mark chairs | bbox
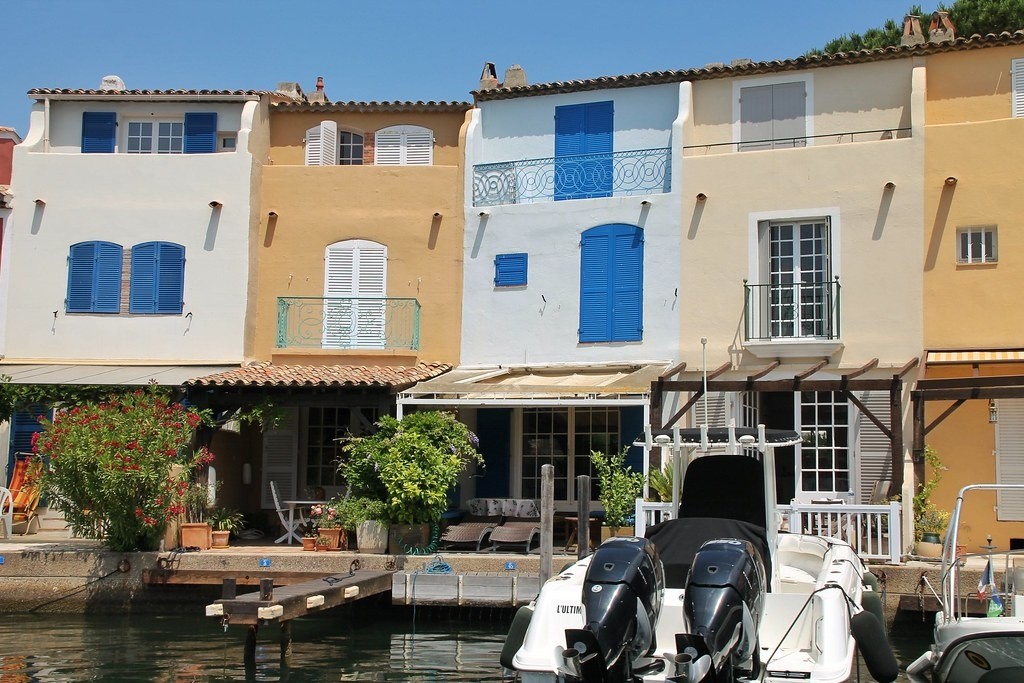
[270,480,310,544]
[2,452,44,536]
[488,521,540,554]
[0,486,12,539]
[438,522,498,553]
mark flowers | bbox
[310,496,344,528]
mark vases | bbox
[317,527,341,551]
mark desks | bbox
[564,517,597,551]
[283,500,328,547]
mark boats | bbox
[499,337,872,683]
[932,483,1024,683]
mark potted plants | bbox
[918,502,951,545]
[333,409,487,553]
[342,493,390,553]
[179,480,224,551]
[202,505,246,549]
[588,437,649,544]
[301,532,317,551]
[315,537,331,551]
